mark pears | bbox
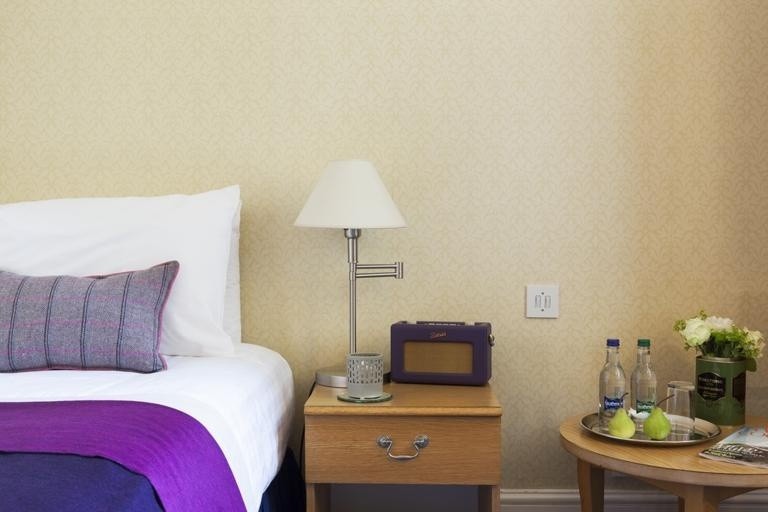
[609,392,636,439]
[643,393,674,440]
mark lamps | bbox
[291,159,410,388]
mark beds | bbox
[0,342,295,512]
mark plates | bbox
[580,413,722,446]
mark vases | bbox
[694,355,747,428]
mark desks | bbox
[559,412,768,512]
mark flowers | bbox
[674,310,765,360]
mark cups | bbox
[665,379,696,437]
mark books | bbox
[698,426,768,469]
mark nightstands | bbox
[303,382,503,512]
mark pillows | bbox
[0,260,180,374]
[0,184,242,356]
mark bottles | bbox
[596,337,659,434]
